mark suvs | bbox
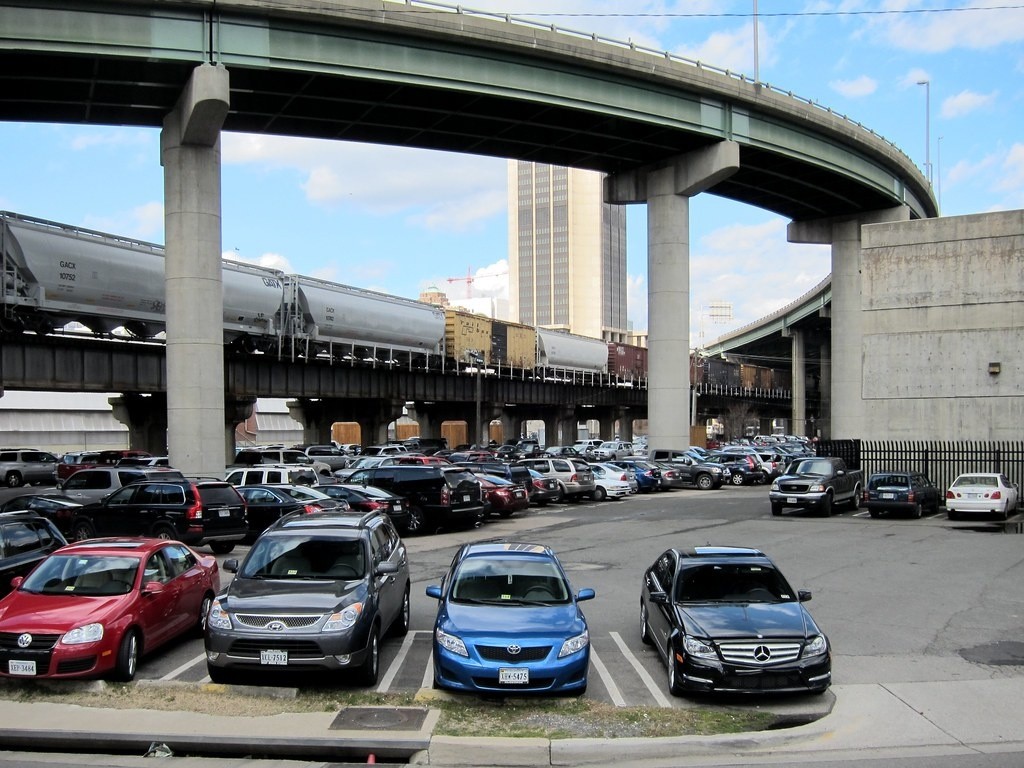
[204,503,412,686]
[0,509,70,599]
[338,463,485,533]
[37,466,186,507]
[517,458,597,503]
[67,476,248,554]
[458,462,534,495]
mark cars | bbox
[0,536,219,682]
[945,472,1019,519]
[529,469,561,507]
[864,472,941,518]
[426,540,595,698]
[0,437,587,483]
[473,473,529,517]
[313,485,411,529]
[588,433,816,500]
[640,543,831,699]
[234,484,350,538]
[1,495,85,516]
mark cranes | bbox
[446,265,508,300]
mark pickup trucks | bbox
[770,456,865,516]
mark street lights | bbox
[918,79,930,180]
